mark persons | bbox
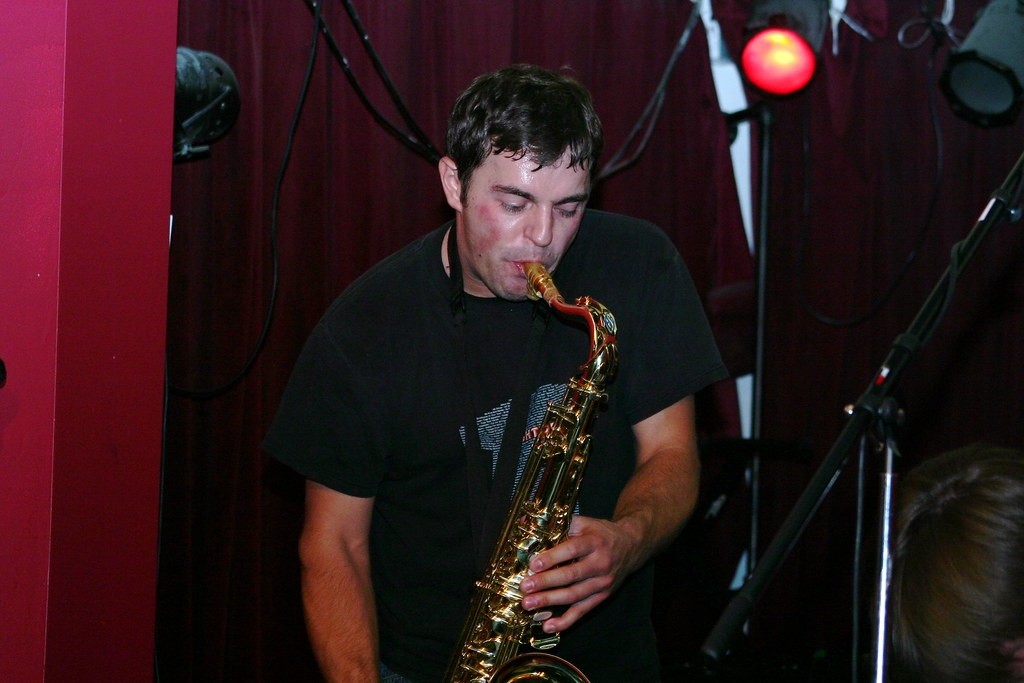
[268,64,722,683]
[890,441,1024,683]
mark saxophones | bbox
[438,263,621,683]
